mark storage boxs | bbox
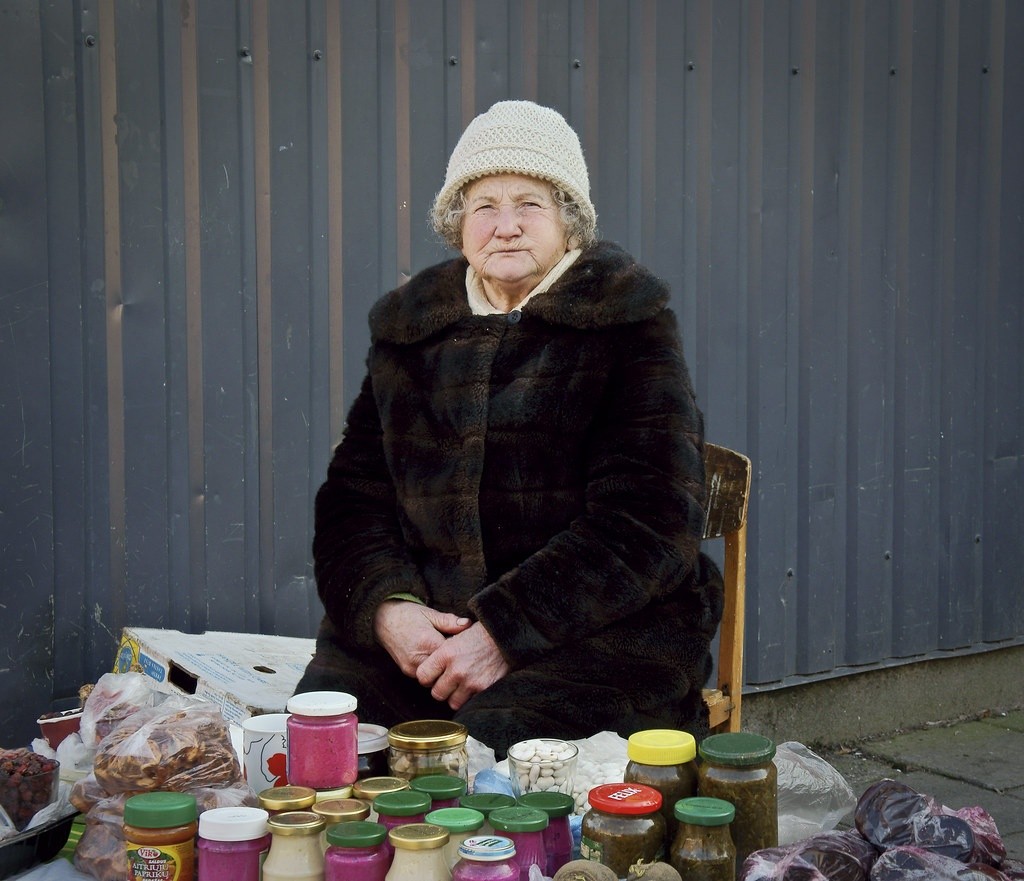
[114,626,315,781]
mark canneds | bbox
[122,692,778,881]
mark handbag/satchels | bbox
[753,741,1014,880]
[52,669,257,877]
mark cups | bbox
[243,713,292,794]
[508,738,579,797]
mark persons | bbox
[281,100,726,754]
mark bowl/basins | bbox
[37,707,83,751]
[0,759,61,832]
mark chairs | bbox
[701,441,752,735]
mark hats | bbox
[430,98,596,249]
